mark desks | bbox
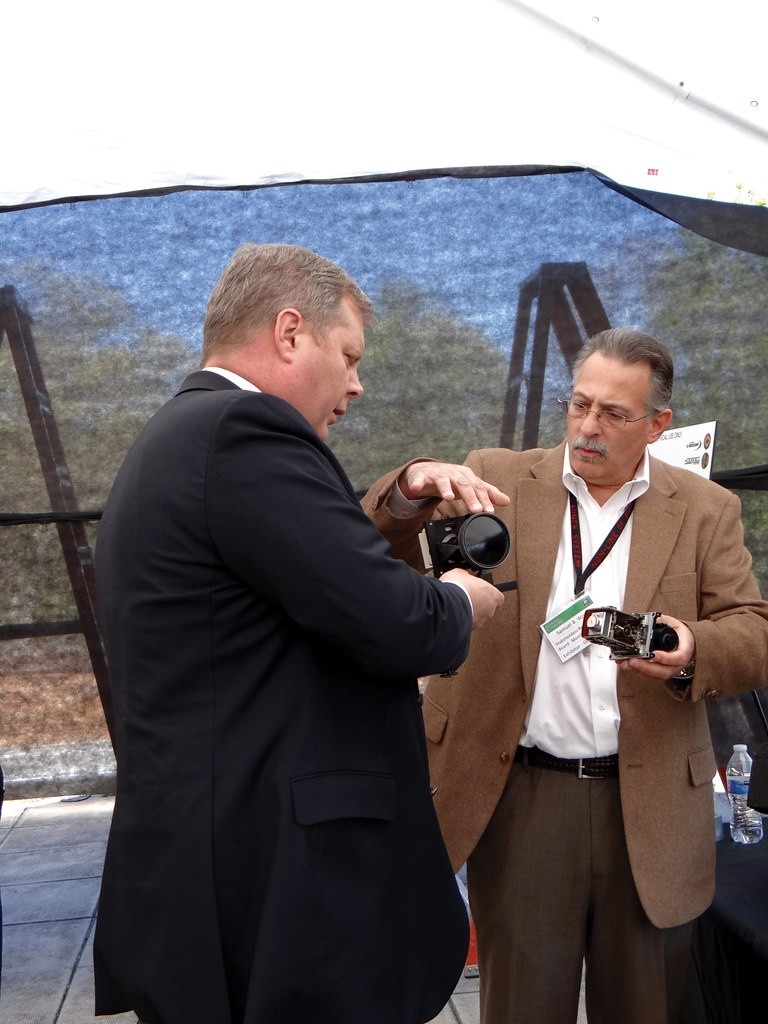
[686,805,768,1024]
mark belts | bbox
[514,746,620,780]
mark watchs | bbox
[676,665,692,678]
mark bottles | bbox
[726,744,763,844]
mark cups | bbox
[715,815,724,841]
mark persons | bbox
[92,242,504,1023]
[361,328,768,1024]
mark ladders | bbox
[2,294,122,783]
[497,256,615,451]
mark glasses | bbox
[557,385,655,430]
